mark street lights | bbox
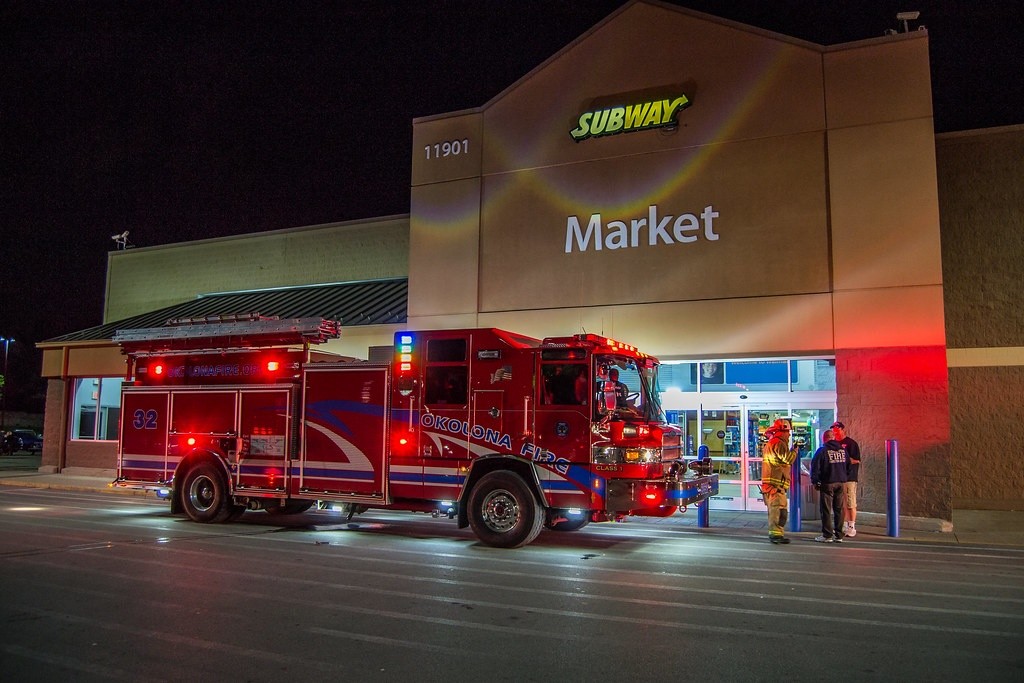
[0,337,15,366]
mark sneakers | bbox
[845,526,856,537]
[815,536,833,543]
[833,526,849,533]
[833,538,842,543]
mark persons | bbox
[701,363,723,384]
[762,420,798,544]
[811,430,847,542]
[830,422,861,537]
[600,368,628,407]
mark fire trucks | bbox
[108,316,720,549]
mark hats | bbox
[830,422,844,429]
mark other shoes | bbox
[770,537,790,544]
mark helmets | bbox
[769,420,792,430]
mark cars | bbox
[1,429,43,453]
